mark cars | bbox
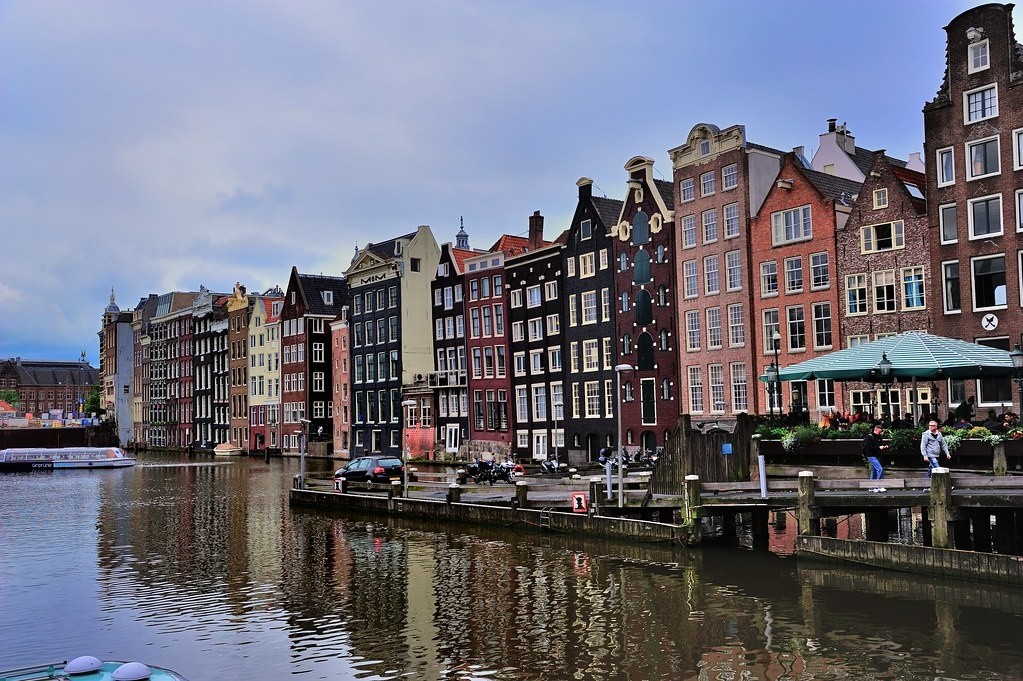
[334,454,404,491]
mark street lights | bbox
[765,359,776,418]
[1008,341,1023,429]
[402,400,417,497]
[878,350,892,429]
[299,418,312,490]
[554,402,565,464]
[616,365,634,510]
[771,330,784,416]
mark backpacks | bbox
[861,434,874,452]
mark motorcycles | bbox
[465,453,518,487]
[600,445,663,472]
[541,453,563,475]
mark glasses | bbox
[929,425,937,427]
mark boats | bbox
[213,443,244,456]
[0,447,138,470]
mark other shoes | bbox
[878,488,887,492]
[869,489,879,492]
[923,488,930,492]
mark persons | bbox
[491,453,496,463]
[861,425,889,493]
[920,420,955,494]
[818,400,1020,434]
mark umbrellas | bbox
[760,331,1015,431]
